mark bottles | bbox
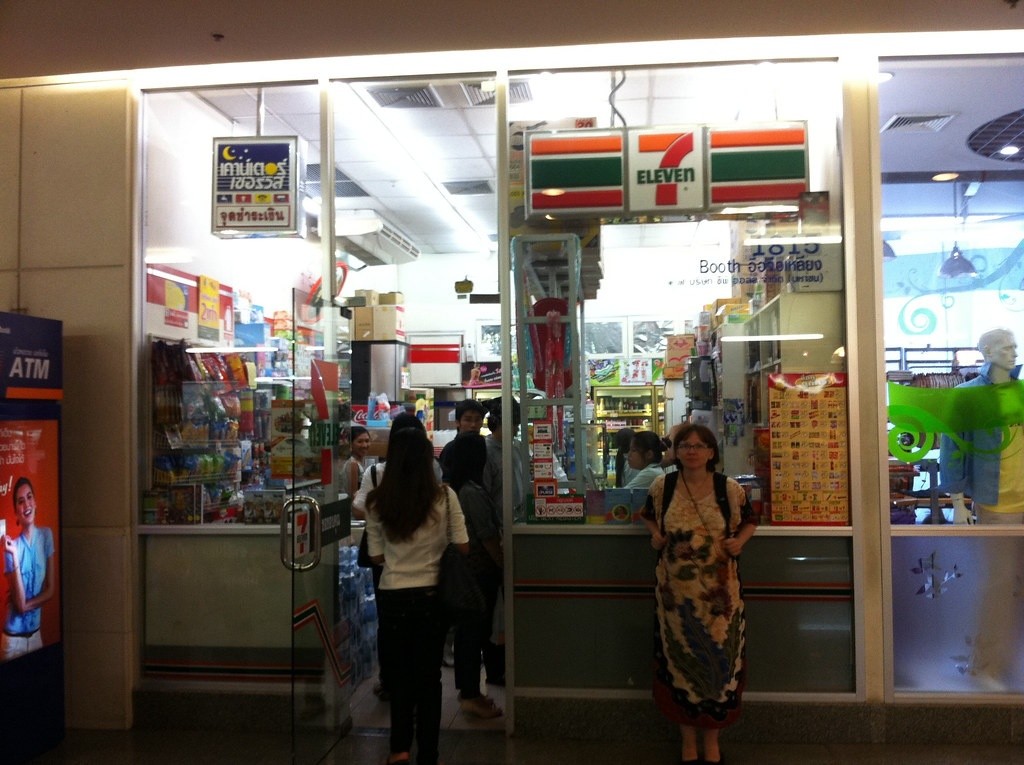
[338,545,379,679]
[599,398,644,410]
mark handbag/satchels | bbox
[358,466,382,568]
[434,543,484,617]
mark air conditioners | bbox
[317,209,420,266]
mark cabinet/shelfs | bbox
[884,347,985,525]
[743,290,849,475]
[709,323,753,478]
[144,332,258,525]
[513,234,587,525]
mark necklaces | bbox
[685,477,706,498]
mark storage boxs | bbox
[379,292,405,305]
[403,387,434,445]
[729,190,844,302]
[355,290,379,306]
[347,306,373,341]
[234,304,316,381]
[374,305,407,343]
[508,115,605,301]
[653,357,665,386]
[664,297,759,378]
[589,359,620,386]
[619,358,652,387]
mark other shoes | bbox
[388,752,409,764]
[443,641,454,666]
[681,750,723,765]
[372,680,389,699]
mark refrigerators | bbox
[589,386,664,483]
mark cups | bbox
[275,385,290,400]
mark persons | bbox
[624,431,672,488]
[443,433,504,718]
[483,396,529,552]
[614,428,675,488]
[939,325,1024,692]
[352,412,442,702]
[640,423,759,765]
[439,399,488,496]
[364,428,469,764]
[342,426,371,493]
[1,476,55,660]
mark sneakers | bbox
[457,692,502,719]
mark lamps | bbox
[883,240,897,263]
[940,183,978,279]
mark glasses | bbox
[678,442,707,452]
